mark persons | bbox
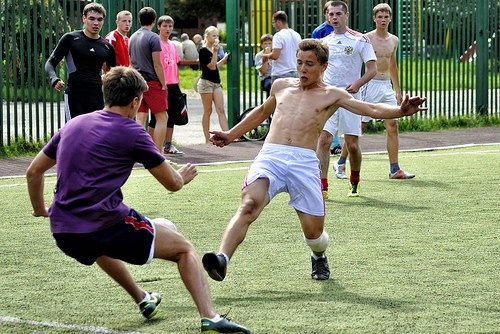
[128,7,173,164]
[193,35,204,51]
[148,15,179,154]
[217,45,224,62]
[268,11,301,82]
[333,3,415,179]
[197,26,241,143]
[316,1,378,199]
[202,39,428,281]
[256,34,274,92]
[45,3,116,121]
[312,1,342,154]
[26,66,250,334]
[181,34,199,60]
[172,37,183,60]
[102,11,132,74]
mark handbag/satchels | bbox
[178,85,189,125]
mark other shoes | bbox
[323,191,329,200]
[330,144,342,156]
[349,181,360,197]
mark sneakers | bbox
[202,251,228,281]
[310,255,330,280]
[333,161,348,179]
[200,313,250,334]
[163,146,184,157]
[139,291,162,318]
[389,169,415,179]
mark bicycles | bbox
[238,106,272,141]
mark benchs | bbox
[178,59,200,66]
